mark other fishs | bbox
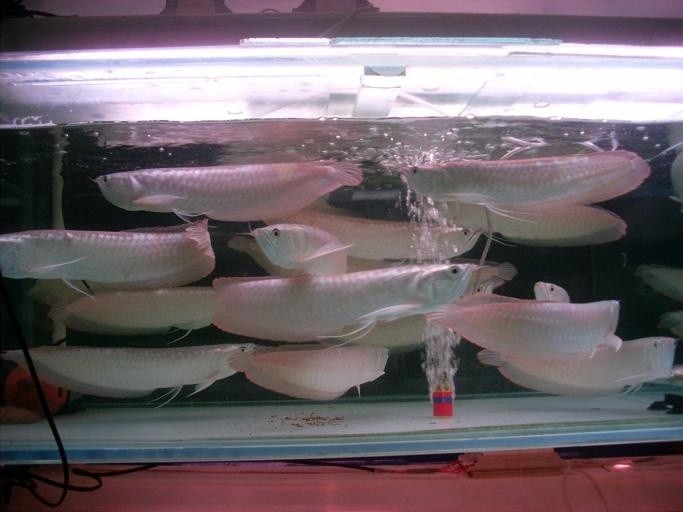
[211,261,518,353]
[93,158,364,230]
